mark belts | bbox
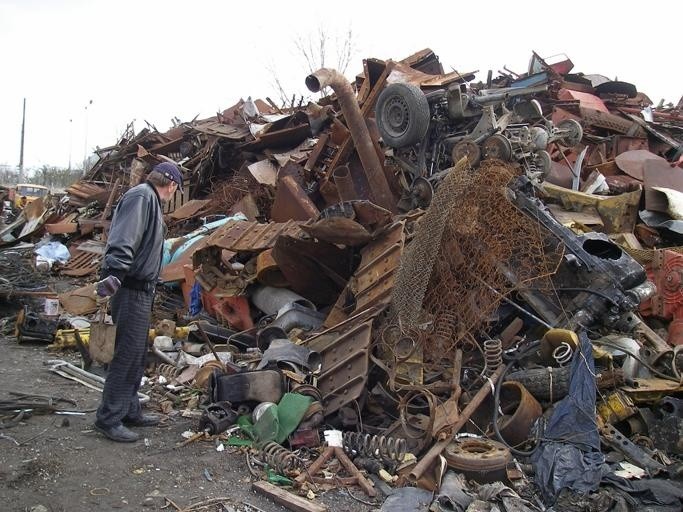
[124,275,158,297]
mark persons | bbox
[91,160,184,443]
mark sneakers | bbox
[93,419,141,443]
[124,409,162,428]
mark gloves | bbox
[92,274,122,305]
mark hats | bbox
[155,160,186,197]
